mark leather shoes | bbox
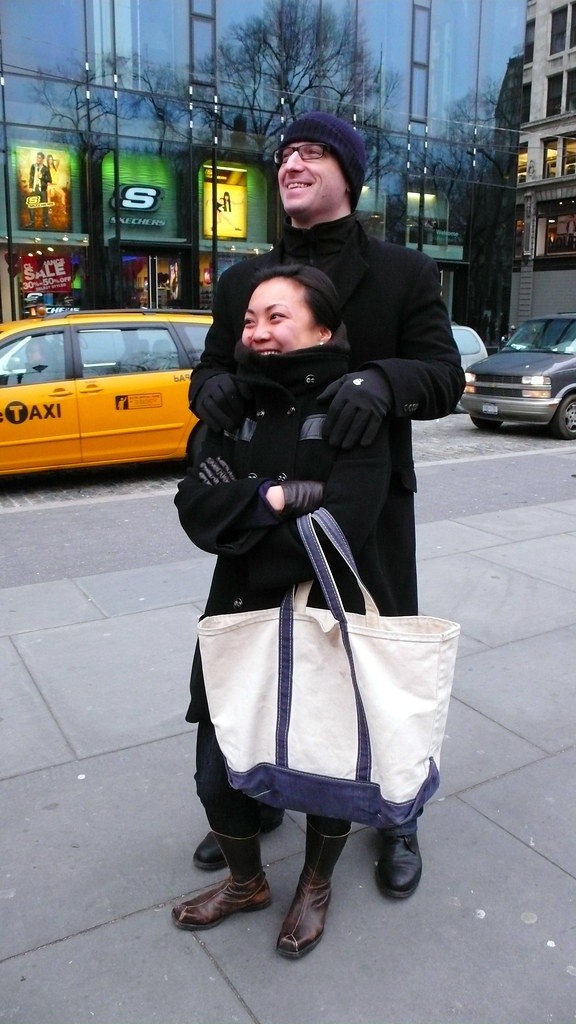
[194,814,284,872]
[375,835,422,898]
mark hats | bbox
[275,112,367,212]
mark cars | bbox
[0,308,213,480]
[449,321,490,416]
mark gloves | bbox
[280,480,327,520]
[316,367,395,452]
[195,373,253,434]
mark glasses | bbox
[274,142,330,165]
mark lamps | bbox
[62,235,68,241]
[83,236,88,242]
[230,245,234,250]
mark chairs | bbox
[113,340,149,372]
[145,339,173,370]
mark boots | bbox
[171,830,271,931]
[276,822,351,960]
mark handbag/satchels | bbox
[197,507,460,829]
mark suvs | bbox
[458,311,575,443]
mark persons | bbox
[187,109,467,901]
[167,264,389,959]
[211,191,243,232]
[24,152,69,229]
